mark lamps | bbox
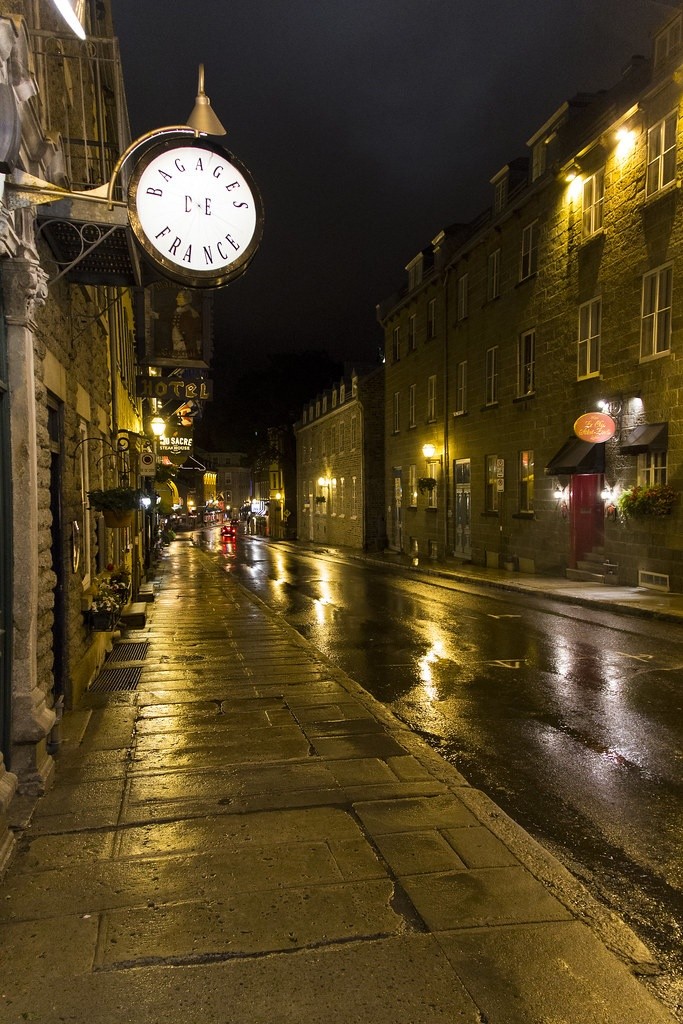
[316,477,330,490]
[276,492,285,504]
[553,487,565,499]
[149,415,166,442]
[185,61,227,138]
[139,488,152,506]
[595,388,642,408]
[422,443,445,473]
[600,487,611,503]
[563,164,580,182]
[152,490,161,505]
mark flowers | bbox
[625,483,677,507]
[85,578,130,614]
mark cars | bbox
[230,518,239,526]
[221,525,236,536]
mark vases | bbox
[92,611,112,629]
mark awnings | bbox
[619,422,668,454]
[545,436,606,475]
[154,480,180,504]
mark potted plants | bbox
[85,487,140,528]
[110,562,130,602]
